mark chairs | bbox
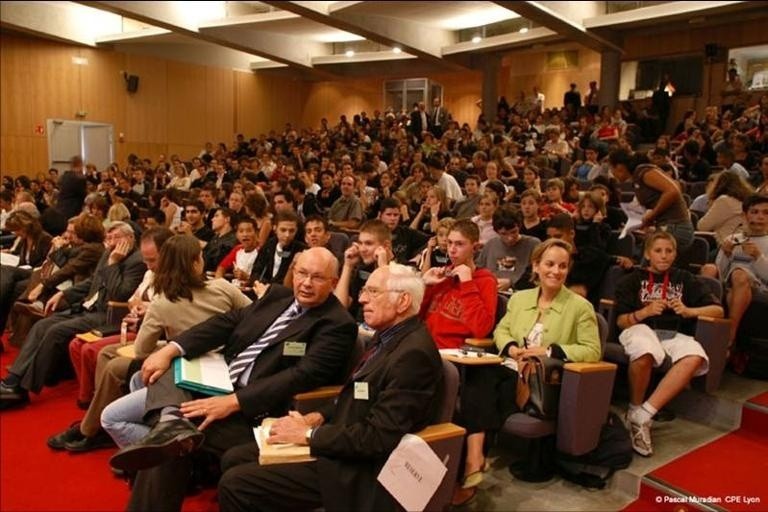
[106,181,733,512]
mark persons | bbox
[0,67,768,512]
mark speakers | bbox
[128,75,138,92]
[705,42,718,61]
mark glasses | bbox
[730,222,755,246]
[293,270,335,284]
[357,287,402,299]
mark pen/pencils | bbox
[523,337,527,349]
[233,261,238,271]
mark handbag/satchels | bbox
[514,354,563,422]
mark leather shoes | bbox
[0,389,30,411]
[12,301,45,319]
[47,427,86,450]
[65,433,116,454]
[461,459,490,489]
[110,418,204,470]
[1,381,25,400]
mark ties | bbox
[228,304,301,382]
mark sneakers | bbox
[626,410,653,456]
